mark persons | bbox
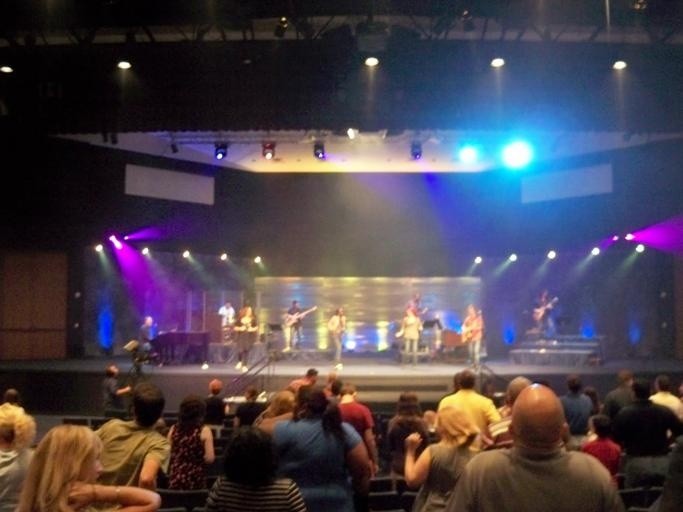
[216,300,236,328]
[327,306,348,368]
[374,372,683,512]
[404,292,426,319]
[239,296,255,319]
[458,304,484,370]
[530,288,558,354]
[136,315,155,353]
[283,301,309,351]
[393,307,422,367]
[232,307,258,373]
[1,364,380,512]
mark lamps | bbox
[213,128,422,160]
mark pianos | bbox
[157,331,207,365]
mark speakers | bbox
[71,217,109,245]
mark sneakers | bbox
[334,362,343,371]
[281,346,290,352]
[235,362,249,373]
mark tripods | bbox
[117,368,158,416]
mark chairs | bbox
[204,415,238,489]
[366,479,418,512]
[156,489,208,512]
[612,472,664,508]
[162,410,180,426]
[62,409,129,432]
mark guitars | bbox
[285,306,318,327]
[533,296,558,321]
[461,319,479,344]
